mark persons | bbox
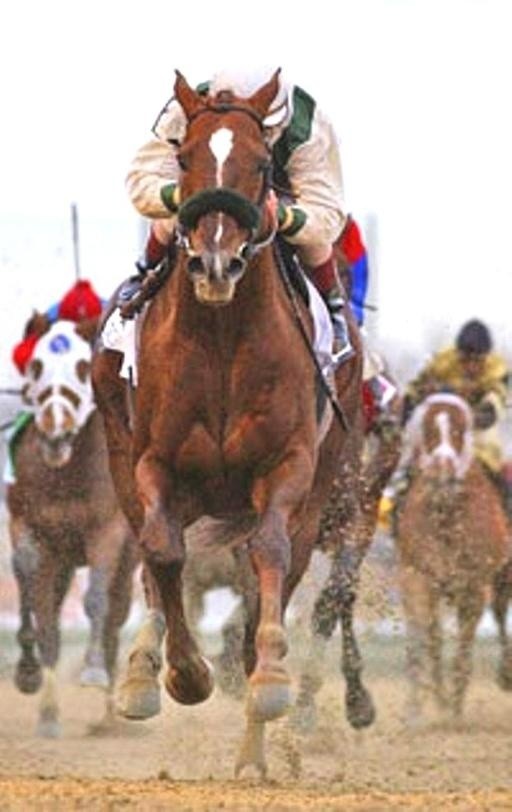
[11,279,509,528]
[125,71,371,330]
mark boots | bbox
[322,283,356,368]
[119,259,156,300]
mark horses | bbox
[90,67,365,790]
[187,369,404,731]
[4,306,133,737]
[387,378,512,733]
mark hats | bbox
[458,320,491,352]
[58,280,103,321]
